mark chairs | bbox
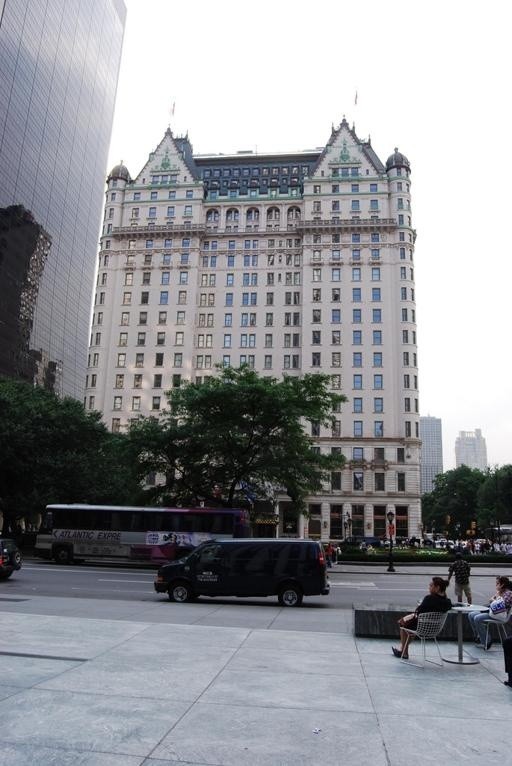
[397,609,445,668]
[482,599,512,650]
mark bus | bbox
[34,503,253,570]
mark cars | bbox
[339,533,469,550]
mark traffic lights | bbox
[445,515,450,525]
[467,530,475,536]
[443,531,448,535]
[471,521,476,528]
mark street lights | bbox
[489,519,495,542]
[456,522,461,553]
[455,527,458,539]
[386,509,396,573]
[344,522,347,541]
[346,518,353,543]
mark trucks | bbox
[497,524,512,544]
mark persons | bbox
[446,552,472,605]
[326,536,511,571]
[467,576,511,650]
[391,577,451,659]
[161,532,192,546]
[501,637,511,687]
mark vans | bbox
[155,538,330,607]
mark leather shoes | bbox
[392,645,408,659]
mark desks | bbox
[442,598,489,664]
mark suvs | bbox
[0,539,25,581]
